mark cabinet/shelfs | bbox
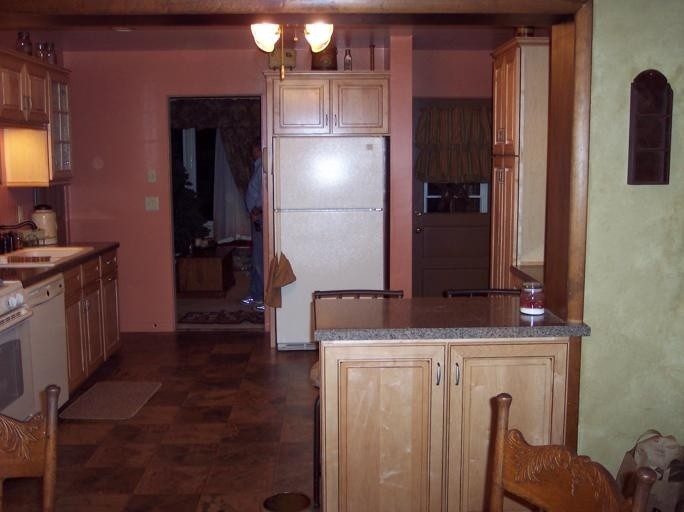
[490,46,549,297]
[325,343,569,512]
[273,79,389,134]
[5,70,74,185]
[65,250,122,393]
[1,52,50,125]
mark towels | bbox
[265,253,297,309]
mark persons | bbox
[238,130,265,313]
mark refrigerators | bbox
[273,136,386,351]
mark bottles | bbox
[343,49,352,71]
[0,204,58,255]
[15,31,58,65]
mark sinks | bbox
[1,255,66,269]
[4,245,94,256]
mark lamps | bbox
[251,23,336,81]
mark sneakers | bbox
[240,296,264,312]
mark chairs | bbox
[489,394,657,512]
[0,385,63,512]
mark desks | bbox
[179,246,238,299]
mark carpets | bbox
[178,310,265,324]
[58,380,163,421]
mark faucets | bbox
[1,219,38,231]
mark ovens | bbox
[0,304,35,422]
